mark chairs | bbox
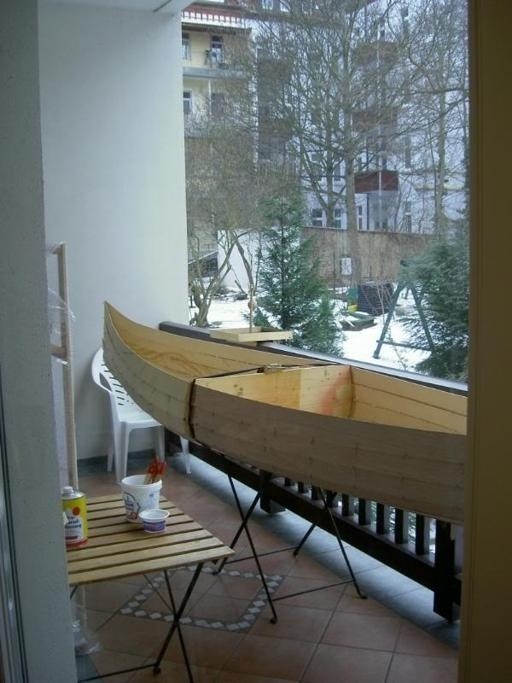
[91,344,191,484]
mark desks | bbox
[66,493,237,682]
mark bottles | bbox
[60,486,89,549]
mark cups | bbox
[120,474,162,524]
[138,508,169,532]
